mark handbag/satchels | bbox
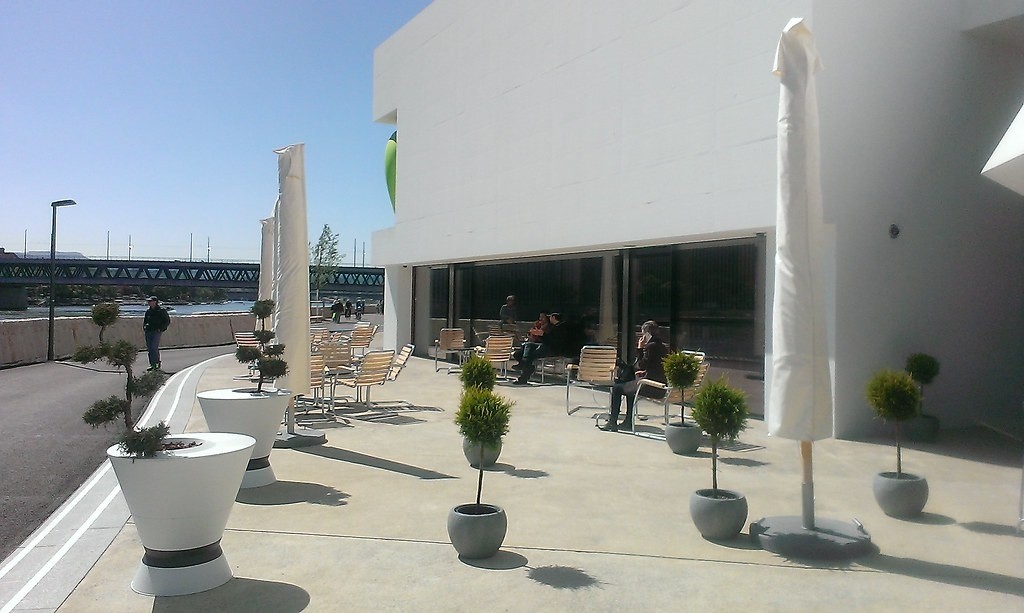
[616,356,637,381]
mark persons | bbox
[142,296,170,371]
[580,306,598,329]
[330,297,384,324]
[597,321,668,432]
[500,296,568,385]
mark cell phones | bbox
[642,332,652,345]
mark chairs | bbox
[232,318,414,429]
[433,328,710,439]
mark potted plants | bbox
[660,348,707,455]
[445,382,516,562]
[866,367,930,519]
[74,299,256,598]
[195,300,292,488]
[902,353,941,441]
[688,372,750,543]
[457,351,503,468]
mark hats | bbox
[145,296,158,301]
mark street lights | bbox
[48,200,75,362]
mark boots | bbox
[617,394,635,431]
[600,388,621,432]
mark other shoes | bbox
[511,361,527,370]
[147,362,161,371]
[514,377,529,385]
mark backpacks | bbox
[158,308,170,332]
